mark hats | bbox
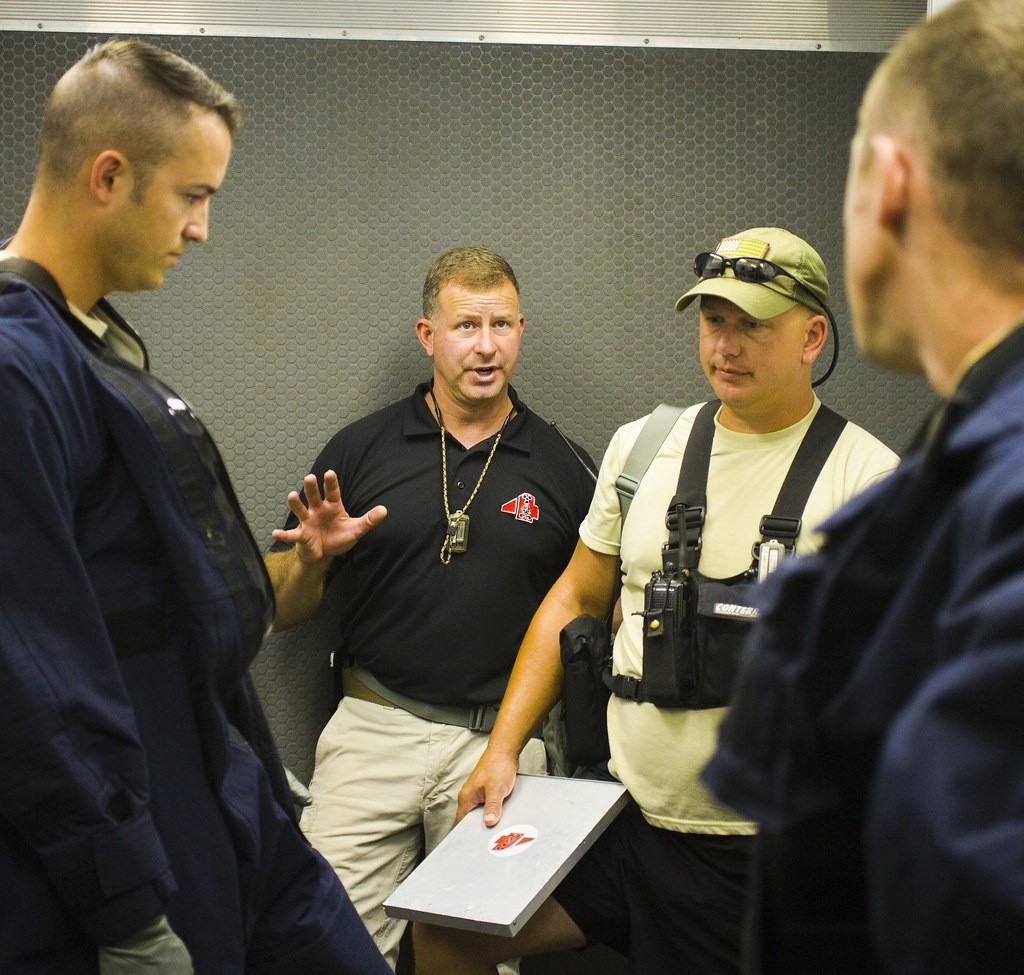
[674,227,830,321]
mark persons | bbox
[697,0,1024,975]
[456,227,901,975]
[2,40,393,975]
[263,248,623,975]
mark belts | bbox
[342,670,545,738]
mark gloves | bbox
[98,914,193,975]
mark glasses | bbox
[693,252,824,306]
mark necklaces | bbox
[430,389,516,565]
